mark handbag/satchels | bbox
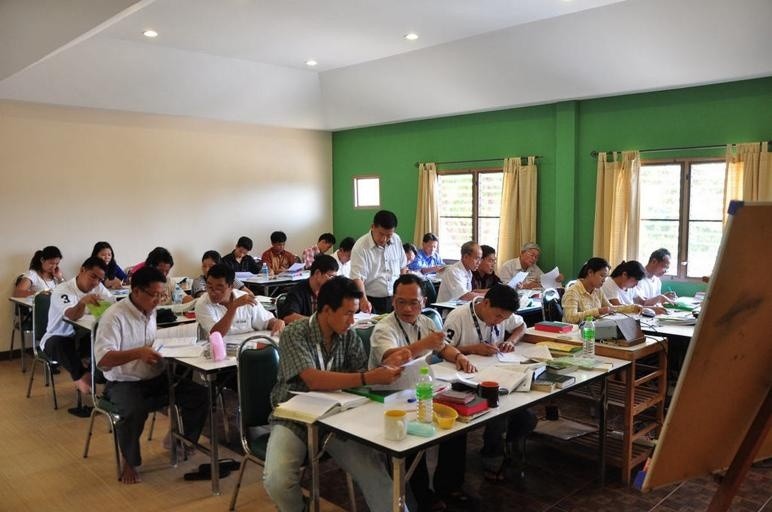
[157,308,177,323]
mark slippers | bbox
[184,458,240,480]
[483,468,508,486]
[68,404,94,417]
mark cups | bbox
[477,381,499,407]
[384,409,407,438]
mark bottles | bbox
[416,367,433,424]
[261,262,270,283]
[582,315,596,358]
[173,285,183,317]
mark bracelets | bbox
[58,278,64,282]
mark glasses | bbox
[139,287,167,300]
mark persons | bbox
[127,231,574,342]
[601,260,676,315]
[442,284,538,493]
[93,265,210,484]
[264,276,410,512]
[350,209,407,314]
[39,258,118,396]
[369,273,483,512]
[634,248,680,304]
[14,247,66,375]
[91,242,127,290]
[561,258,643,325]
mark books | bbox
[86,298,113,319]
[272,340,582,427]
[153,323,202,360]
[653,291,706,327]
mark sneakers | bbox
[425,490,474,512]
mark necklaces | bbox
[39,273,57,288]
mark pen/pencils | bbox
[667,286,672,294]
[377,364,407,376]
[156,345,163,352]
[429,327,452,344]
[484,341,504,357]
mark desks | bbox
[9,258,722,512]
